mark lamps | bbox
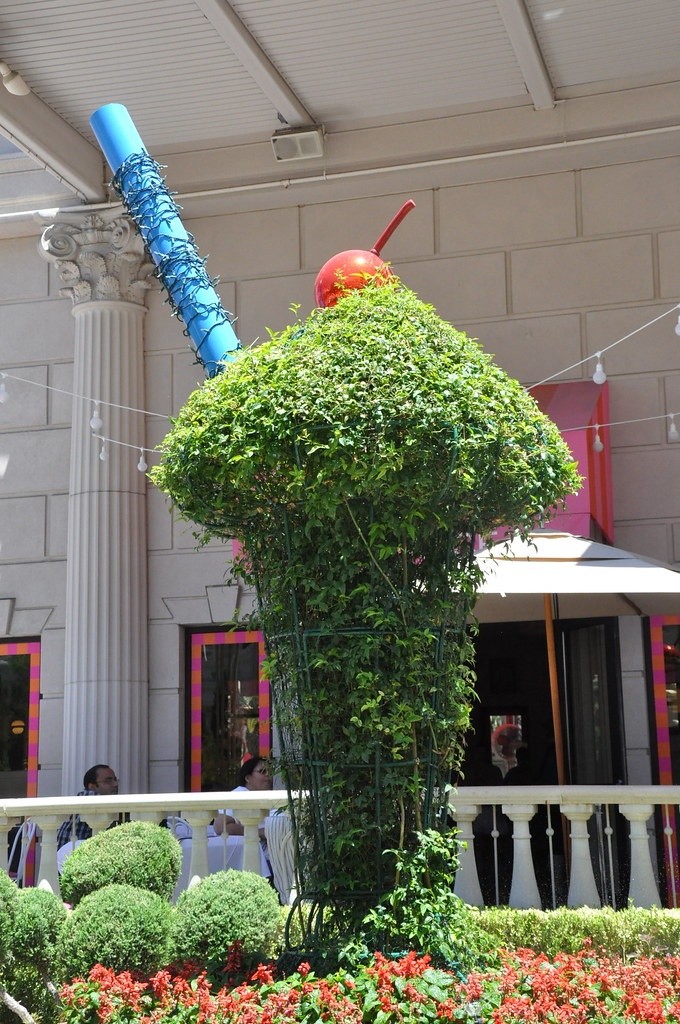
[272,124,325,162]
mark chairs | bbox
[266,814,315,907]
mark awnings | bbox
[230,380,615,578]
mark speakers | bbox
[270,125,324,162]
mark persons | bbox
[58,763,132,877]
[213,756,276,877]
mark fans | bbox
[492,723,528,769]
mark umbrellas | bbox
[415,524,680,883]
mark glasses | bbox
[253,769,273,775]
[94,777,120,784]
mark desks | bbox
[56,823,273,908]
